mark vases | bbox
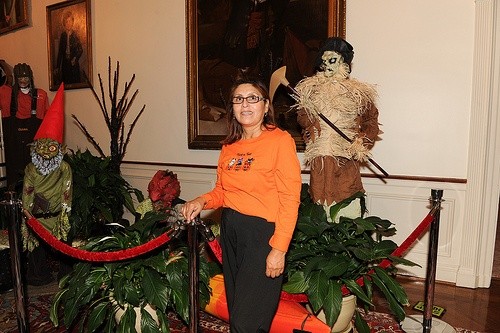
[108,288,159,333]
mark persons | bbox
[181,80,302,333]
[295,38,379,224]
[0,63,73,286]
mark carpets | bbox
[0,283,484,333]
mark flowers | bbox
[51,170,212,333]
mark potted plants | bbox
[279,183,423,333]
[63,147,145,248]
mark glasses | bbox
[230,95,265,103]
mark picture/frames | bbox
[46,0,94,91]
[0,0,29,34]
[185,0,346,152]
